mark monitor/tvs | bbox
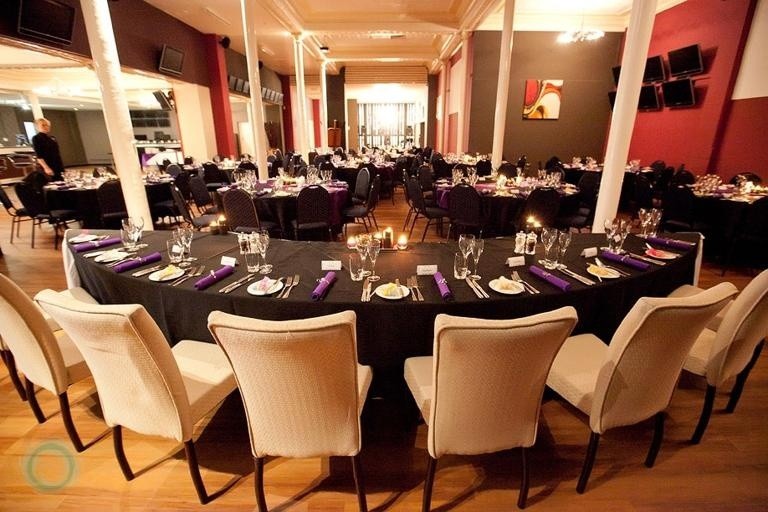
[611,65,621,88]
[608,92,616,112]
[237,79,243,91]
[643,55,666,83]
[661,78,696,108]
[262,88,283,102]
[637,85,660,110]
[667,43,704,78]
[228,75,236,90]
[152,90,173,112]
[16,0,77,49]
[157,42,187,77]
[243,81,249,92]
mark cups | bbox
[244,247,259,273]
[348,253,364,281]
[453,253,467,280]
[608,232,620,255]
[166,239,183,264]
[544,245,558,270]
[120,228,136,248]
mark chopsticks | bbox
[630,253,666,265]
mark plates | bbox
[586,267,620,279]
[488,278,524,295]
[247,279,283,296]
[149,270,185,282]
[645,250,677,259]
[94,253,128,263]
[375,284,409,300]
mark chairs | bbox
[219,146,530,178]
[165,166,181,179]
[33,289,238,505]
[223,190,270,232]
[204,168,219,191]
[445,183,482,239]
[14,183,63,248]
[409,187,448,242]
[0,286,101,402]
[404,306,579,512]
[545,281,740,494]
[682,268,768,446]
[190,178,214,213]
[347,167,372,229]
[343,175,381,235]
[403,181,440,236]
[516,187,560,232]
[667,284,764,414]
[171,185,218,231]
[30,190,86,251]
[208,310,372,512]
[214,143,528,179]
[0,273,112,453]
[0,186,30,246]
[293,185,332,241]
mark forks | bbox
[595,258,631,275]
[514,270,540,294]
[595,261,627,278]
[406,278,417,301]
[411,275,424,301]
[108,257,141,268]
[511,275,534,294]
[107,252,137,266]
[276,276,292,298]
[646,242,652,249]
[282,274,299,298]
[168,266,196,286]
[173,265,205,286]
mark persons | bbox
[32,117,65,176]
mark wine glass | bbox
[468,239,483,281]
[60,170,77,184]
[458,234,475,275]
[257,234,273,274]
[173,230,184,258]
[600,217,619,249]
[122,217,140,251]
[651,208,661,238]
[538,227,557,265]
[636,209,646,237]
[557,230,570,269]
[450,167,479,187]
[233,170,257,189]
[132,217,148,248]
[307,167,334,185]
[357,238,371,276]
[617,218,633,255]
[367,240,381,282]
[642,213,651,237]
[179,228,198,261]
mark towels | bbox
[310,270,336,301]
[433,271,451,300]
[645,237,691,252]
[193,265,234,290]
[113,251,162,272]
[256,190,269,196]
[600,250,650,271]
[73,237,121,253]
[529,265,571,291]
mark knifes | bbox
[558,268,592,286]
[472,279,489,299]
[225,276,255,293]
[367,283,372,301]
[564,269,596,284]
[219,273,255,293]
[465,277,483,298]
[361,279,368,302]
